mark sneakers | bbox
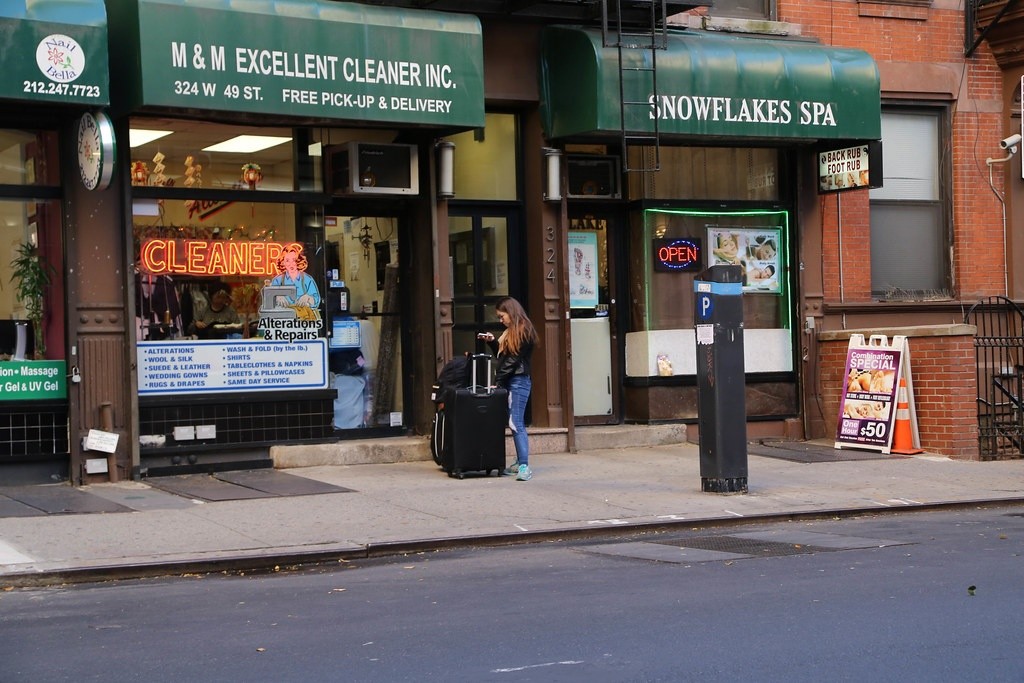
[504,460,519,475]
[516,464,532,481]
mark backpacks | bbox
[431,352,472,407]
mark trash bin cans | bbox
[45,453,70,483]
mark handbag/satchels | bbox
[430,408,445,465]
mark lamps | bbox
[434,138,456,199]
[541,146,564,203]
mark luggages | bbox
[444,353,508,477]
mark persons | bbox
[713,231,778,286]
[819,171,868,191]
[477,297,539,481]
[844,368,887,420]
[187,282,246,340]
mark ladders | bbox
[616,0,661,175]
